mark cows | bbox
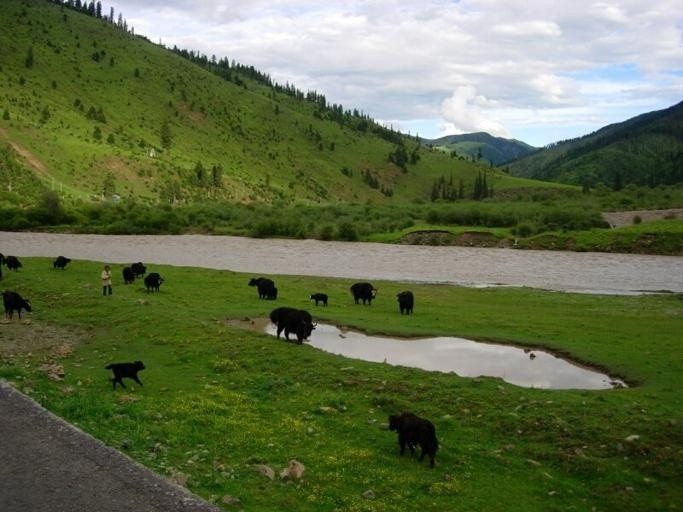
[396,291,414,315]
[270,306,317,344]
[104,360,146,390]
[247,277,277,300]
[388,411,438,469]
[1,291,32,320]
[53,255,71,270]
[0,253,6,265]
[123,262,164,293]
[310,293,328,307]
[5,256,22,272]
[350,282,379,305]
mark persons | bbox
[101,265,113,297]
